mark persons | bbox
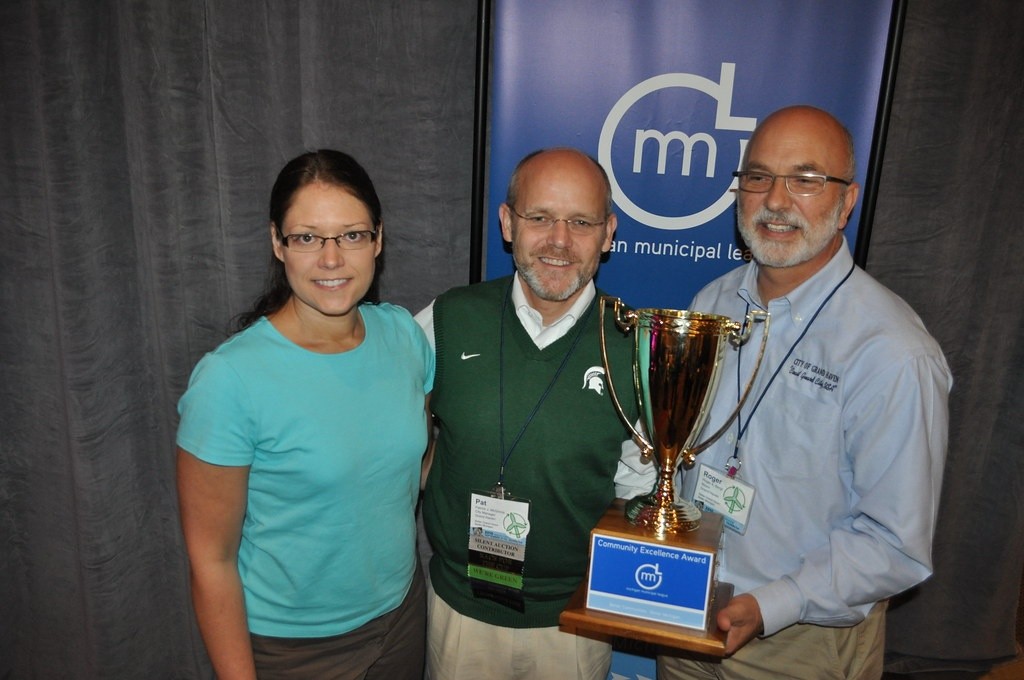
[174,149,437,680]
[615,105,953,679]
[411,151,657,680]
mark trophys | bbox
[560,296,770,661]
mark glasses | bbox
[512,205,607,235]
[731,169,853,196]
[274,229,378,251]
